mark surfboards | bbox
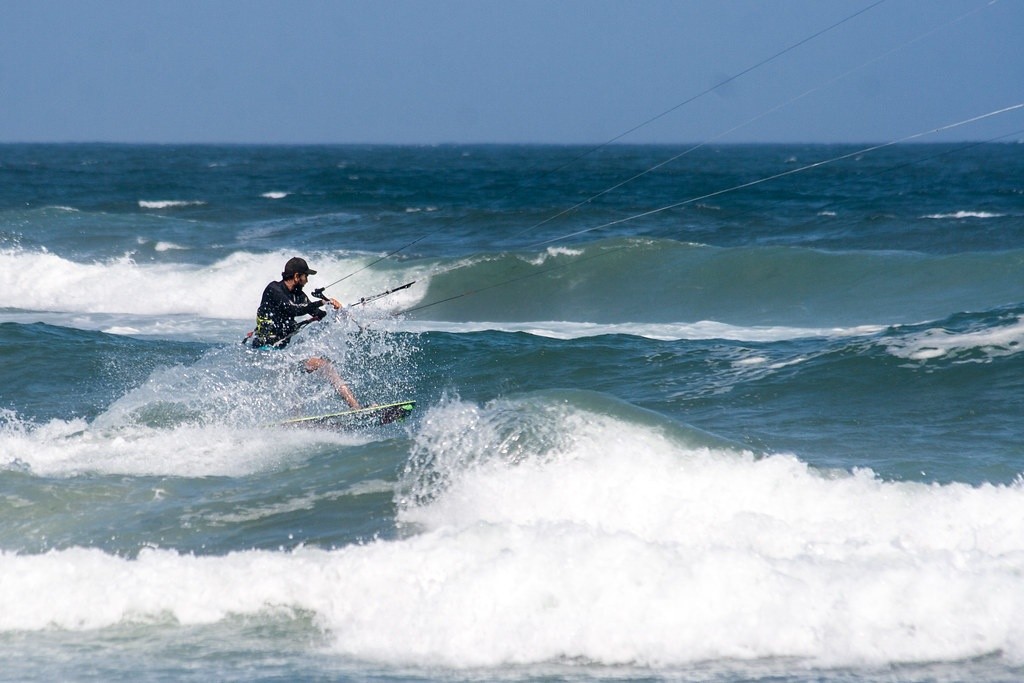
[249,400,418,436]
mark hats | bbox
[284,256,317,275]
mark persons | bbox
[253,257,364,413]
